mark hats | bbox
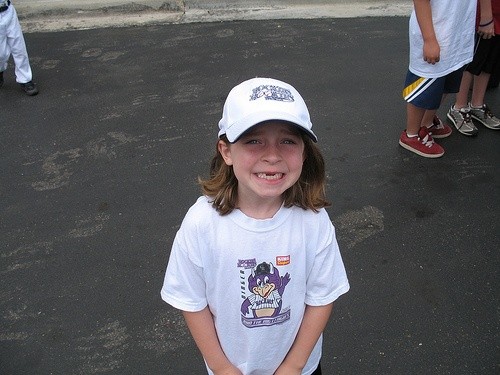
[218,77,318,144]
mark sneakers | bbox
[467,102,500,130]
[20,80,39,96]
[446,104,479,136]
[398,126,446,159]
[419,115,452,138]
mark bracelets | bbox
[478,18,494,28]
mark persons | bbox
[161,79,350,375]
[399,0,477,158]
[0,0,38,96]
[446,1,500,135]
